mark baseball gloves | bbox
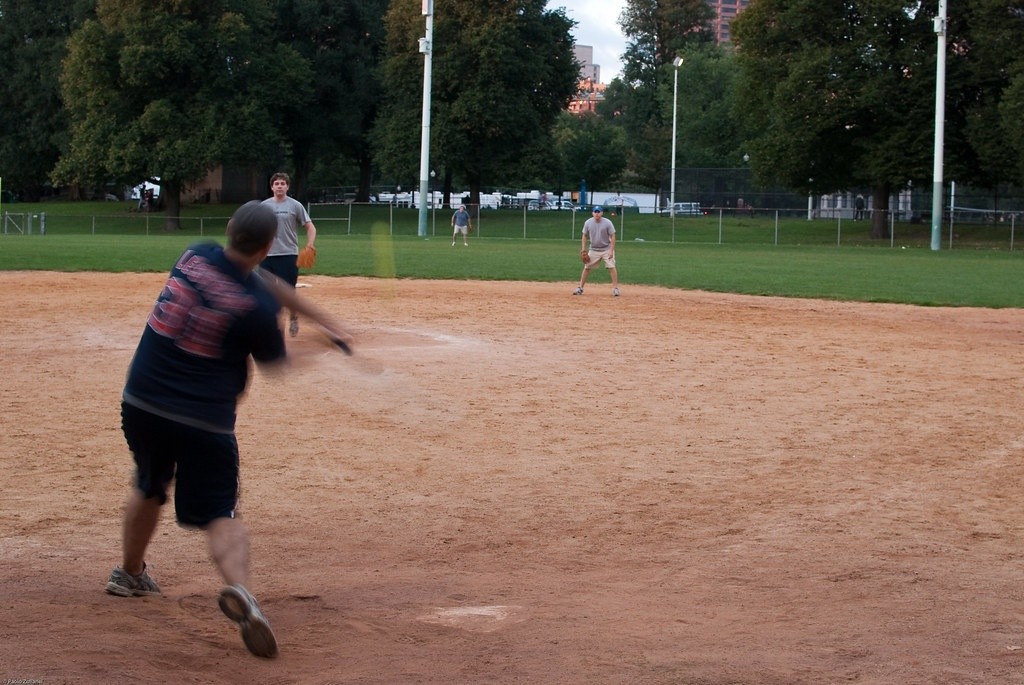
[581,250,591,264]
[297,245,317,270]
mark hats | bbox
[592,206,603,212]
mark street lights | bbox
[669,56,684,219]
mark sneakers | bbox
[573,287,583,295]
[452,242,456,246]
[464,242,469,247]
[612,288,620,296]
[288,316,299,337]
[217,583,280,659]
[104,560,162,597]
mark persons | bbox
[104,201,351,659]
[854,193,864,220]
[260,172,316,337]
[572,206,620,296]
[450,204,473,247]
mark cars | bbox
[528,200,586,211]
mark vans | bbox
[659,202,702,215]
